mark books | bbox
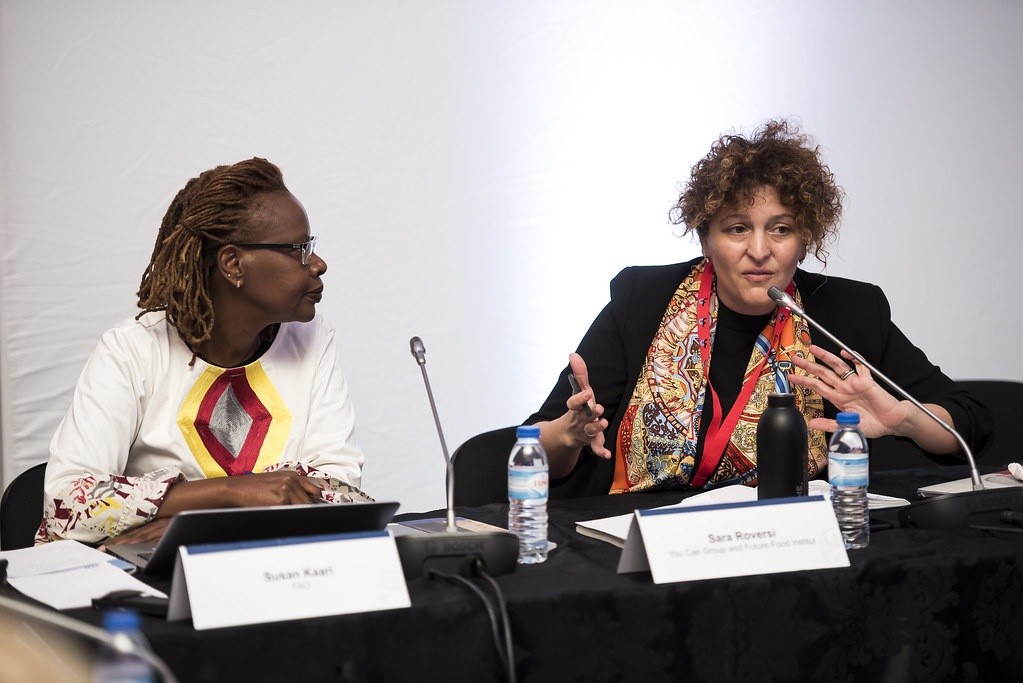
[575,505,670,550]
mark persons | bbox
[34,156,366,552]
[523,121,995,500]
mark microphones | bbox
[767,285,1023,527]
[385,338,521,582]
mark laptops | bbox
[105,502,400,578]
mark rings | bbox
[840,369,854,379]
[584,423,596,436]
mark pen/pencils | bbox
[568,373,593,416]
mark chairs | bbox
[446,423,519,509]
[0,462,49,552]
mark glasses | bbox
[215,235,317,266]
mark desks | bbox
[1,463,1023,683]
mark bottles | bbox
[756,393,808,501]
[828,413,870,549]
[96,613,154,683]
[507,426,549,563]
